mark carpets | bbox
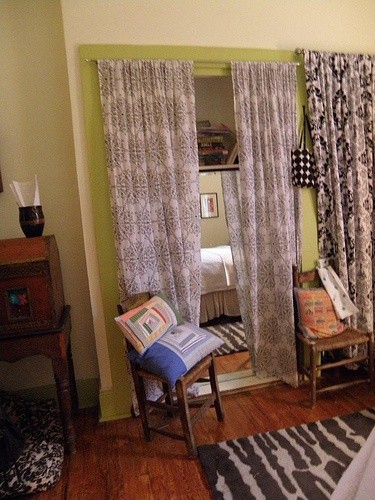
[204,322,247,358]
[196,408,375,500]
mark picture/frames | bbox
[199,192,219,219]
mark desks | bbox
[0,305,80,456]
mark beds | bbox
[199,245,240,322]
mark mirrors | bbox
[98,171,296,406]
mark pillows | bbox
[292,287,350,339]
[126,321,226,390]
[113,291,185,357]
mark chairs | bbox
[117,290,226,460]
[292,259,374,409]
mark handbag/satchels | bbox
[291,115,320,188]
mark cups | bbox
[19,205,45,238]
